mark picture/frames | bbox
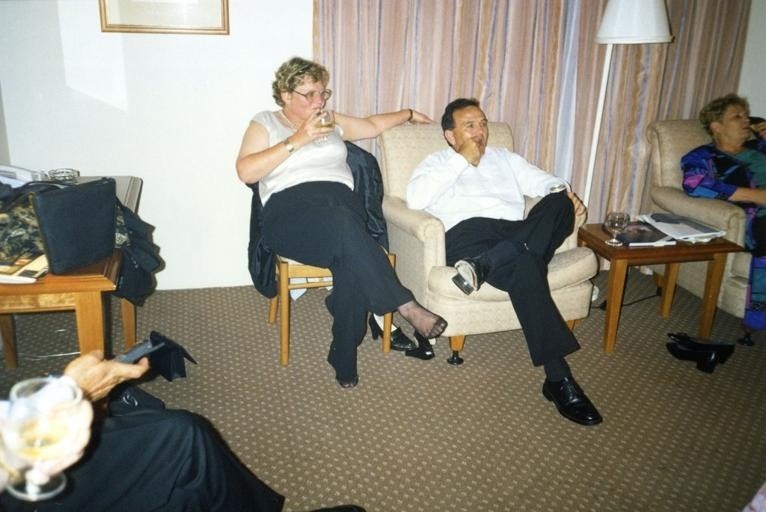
[96,0,232,36]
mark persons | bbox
[0,348,365,512]
[680,96,766,253]
[406,98,603,426]
[235,57,448,388]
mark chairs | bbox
[251,139,398,369]
[641,114,764,347]
[365,120,599,364]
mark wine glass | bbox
[602,209,628,247]
[5,378,85,501]
[308,107,337,146]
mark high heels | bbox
[369,313,433,359]
[667,332,735,372]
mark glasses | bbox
[292,89,331,101]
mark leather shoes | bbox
[543,378,602,425]
[452,256,484,295]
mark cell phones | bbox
[116,341,165,363]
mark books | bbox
[0,253,49,285]
[641,211,727,242]
[601,219,678,247]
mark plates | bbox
[48,167,80,182]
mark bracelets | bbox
[407,109,413,122]
[283,138,294,153]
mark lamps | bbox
[581,2,676,231]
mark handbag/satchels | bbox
[32,178,116,275]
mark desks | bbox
[576,219,745,357]
[0,172,143,367]
